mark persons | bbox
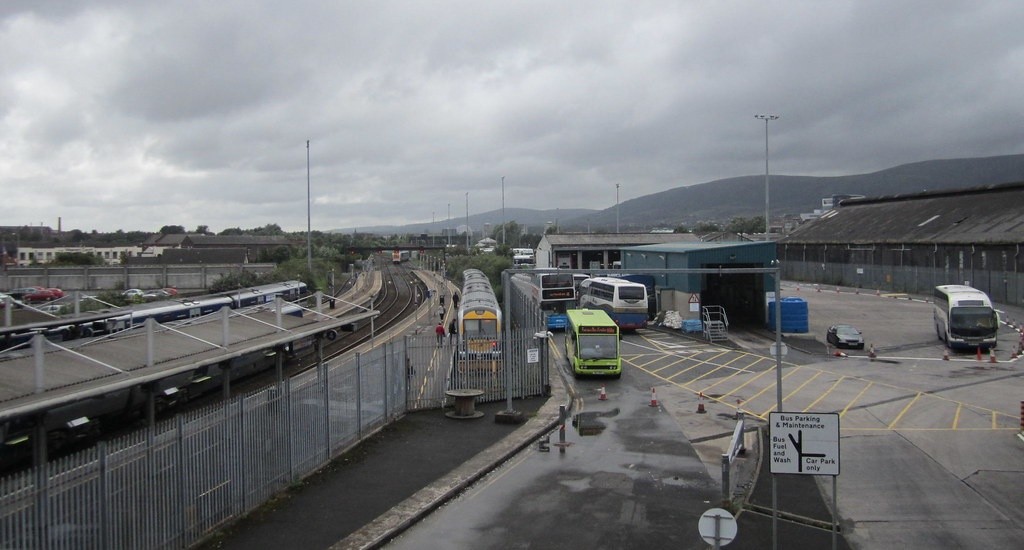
[449,319,458,344]
[437,302,446,323]
[435,322,446,348]
[452,292,459,308]
[407,359,416,394]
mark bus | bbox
[565,308,622,378]
[576,276,649,333]
[511,269,575,330]
[933,284,1000,352]
[513,249,535,269]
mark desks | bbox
[444,389,484,418]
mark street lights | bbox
[754,114,779,241]
[501,175,506,243]
[616,182,621,232]
[465,191,469,251]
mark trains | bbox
[456,268,503,376]
[392,251,410,264]
[13,279,307,342]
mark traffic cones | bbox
[733,399,743,419]
[648,389,659,407]
[835,351,848,357]
[976,347,982,359]
[942,346,949,361]
[598,384,608,401]
[868,343,876,357]
[988,347,999,363]
[695,393,708,413]
[1010,345,1018,358]
[1005,316,1024,355]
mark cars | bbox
[827,324,864,349]
[122,288,177,298]
[0,286,64,308]
